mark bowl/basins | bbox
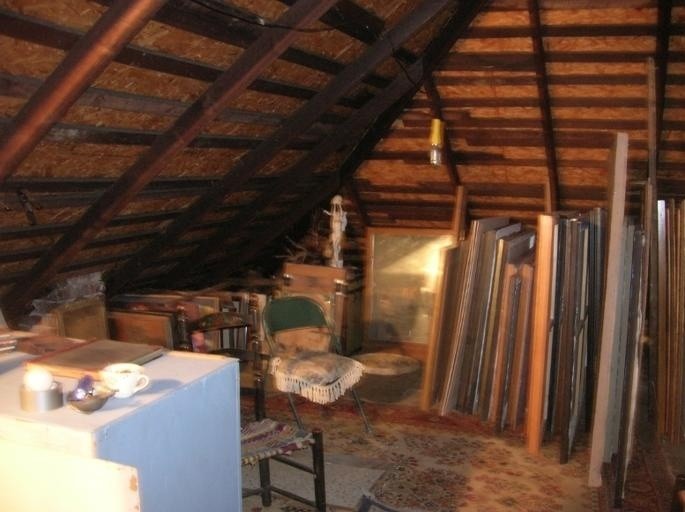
[63,385,115,413]
[350,352,421,403]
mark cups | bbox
[101,363,149,398]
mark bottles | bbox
[190,322,205,352]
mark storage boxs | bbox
[276,260,364,355]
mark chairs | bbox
[261,295,374,438]
[169,308,327,512]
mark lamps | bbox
[428,119,446,165]
